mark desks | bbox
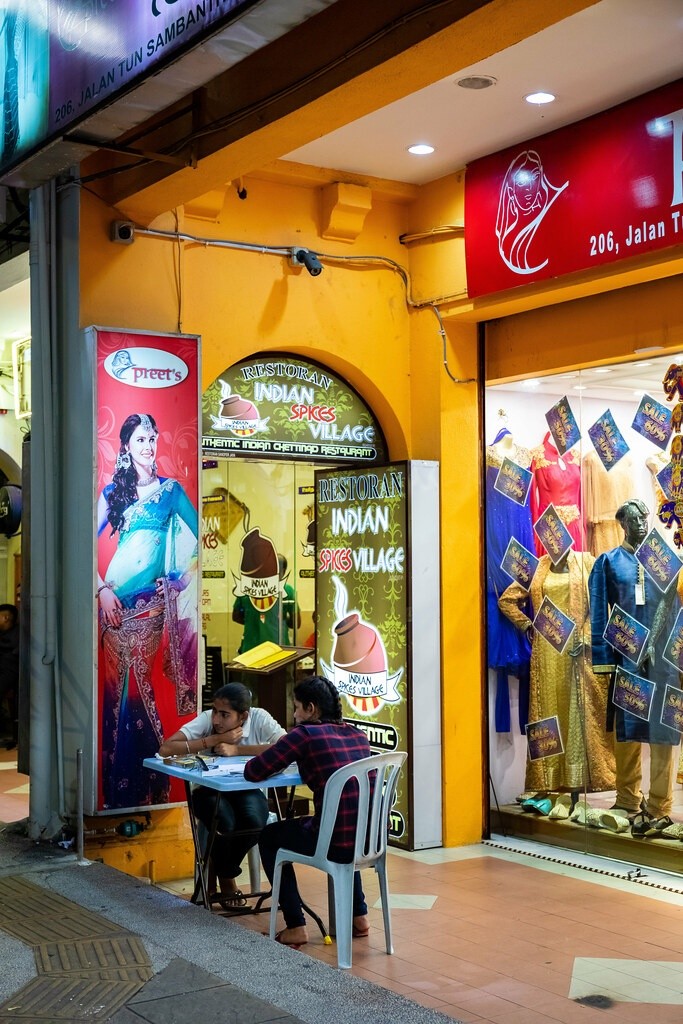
[142,755,306,917]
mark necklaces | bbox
[136,472,156,486]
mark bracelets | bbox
[98,584,108,592]
[186,740,191,754]
[201,737,208,749]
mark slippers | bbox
[353,925,369,937]
[261,930,301,949]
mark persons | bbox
[243,676,391,951]
[486,426,683,821]
[0,603,18,750]
[97,414,197,808]
[159,683,288,908]
[304,610,317,647]
[232,554,302,654]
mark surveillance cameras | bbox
[302,254,324,277]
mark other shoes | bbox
[516,789,683,840]
[218,890,247,906]
[208,885,217,900]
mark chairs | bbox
[192,811,277,910]
[269,752,409,968]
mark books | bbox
[232,641,299,670]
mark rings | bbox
[112,609,118,614]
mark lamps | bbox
[287,246,324,276]
